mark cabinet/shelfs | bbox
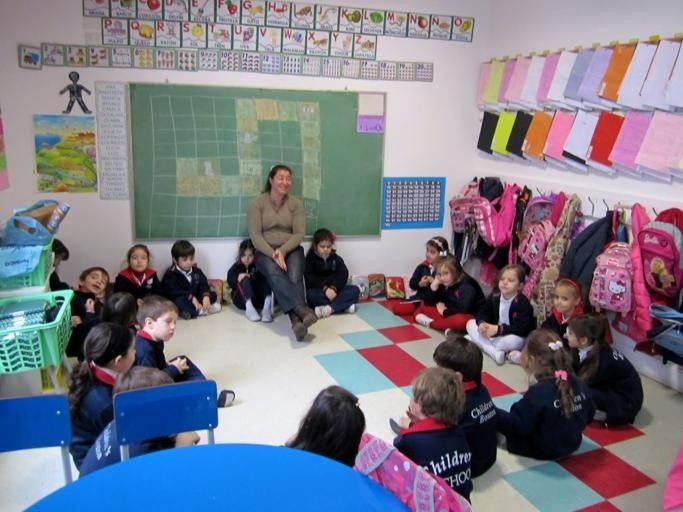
[0,268,55,397]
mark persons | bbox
[227,239,273,323]
[393,236,449,316]
[413,255,485,331]
[78,366,201,482]
[282,385,366,469]
[565,313,644,428]
[160,240,221,320]
[131,295,189,382]
[432,337,498,479]
[392,366,473,507]
[114,244,160,298]
[505,278,586,369]
[49,238,73,291]
[68,321,137,472]
[496,327,596,461]
[83,295,132,334]
[305,228,360,319]
[247,165,318,343]
[444,264,536,365]
[69,267,109,351]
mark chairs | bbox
[0,394,73,486]
[113,381,219,462]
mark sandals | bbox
[216,390,235,409]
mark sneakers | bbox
[261,308,273,321]
[199,302,223,316]
[492,349,505,365]
[445,328,458,339]
[507,349,521,365]
[314,304,332,318]
[415,312,435,328]
[245,306,261,322]
[389,417,406,434]
[291,303,318,341]
[345,304,356,314]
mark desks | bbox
[21,444,407,511]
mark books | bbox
[541,109,576,171]
[596,44,635,101]
[561,109,600,175]
[518,55,545,112]
[520,111,554,169]
[662,38,683,110]
[616,41,657,112]
[536,54,563,112]
[575,47,619,110]
[633,110,683,185]
[476,109,497,161]
[503,57,531,113]
[585,110,626,178]
[546,51,579,113]
[667,133,683,181]
[562,49,594,112]
[477,63,495,114]
[607,111,652,180]
[639,37,681,113]
[483,60,505,112]
[506,110,535,166]
[491,110,517,163]
[498,60,522,110]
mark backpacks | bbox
[588,206,633,319]
[517,191,568,271]
[471,182,520,250]
[354,435,473,512]
[636,208,683,299]
[447,183,480,233]
[647,303,683,366]
[521,192,555,229]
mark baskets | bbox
[0,286,76,376]
[0,234,54,285]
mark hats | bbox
[572,314,605,343]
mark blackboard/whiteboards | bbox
[122,80,388,245]
